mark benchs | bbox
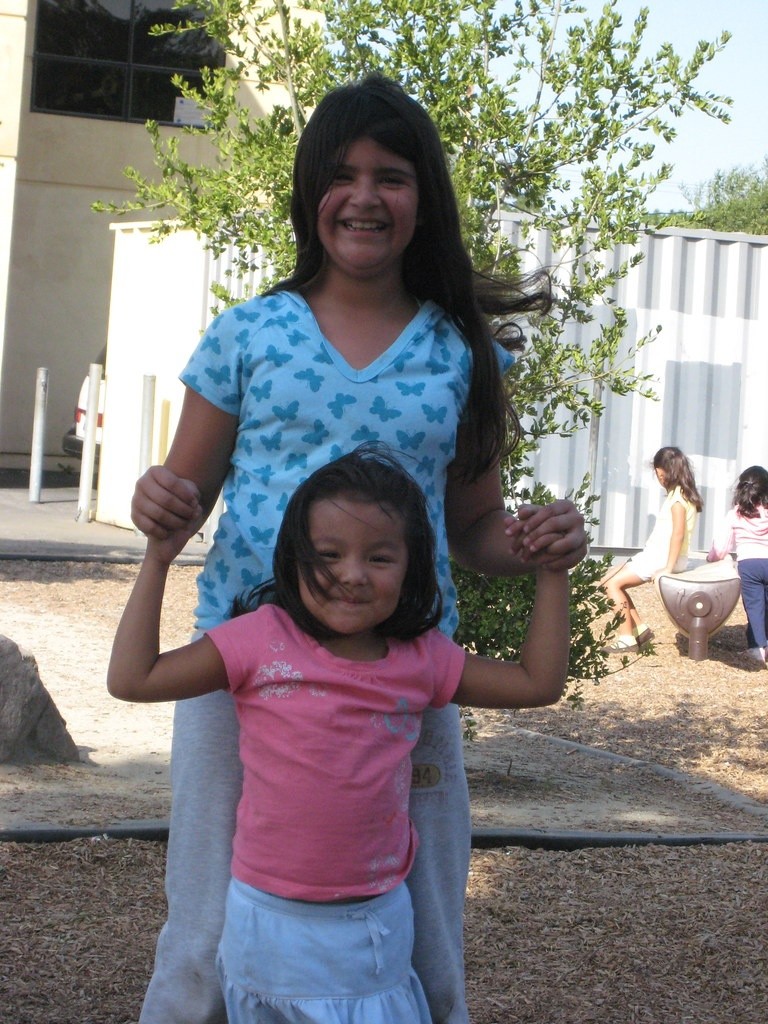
[659,556,741,661]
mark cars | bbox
[62,343,107,461]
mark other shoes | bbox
[635,628,655,647]
[737,647,767,667]
[600,641,639,653]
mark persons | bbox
[705,465,768,664]
[108,73,586,1024]
[600,447,706,651]
